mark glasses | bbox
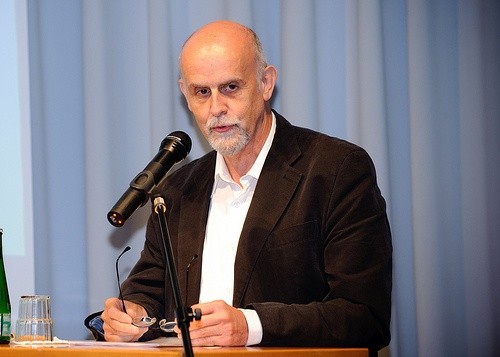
[116,246,198,332]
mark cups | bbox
[15,296,52,344]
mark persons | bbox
[83,21,393,357]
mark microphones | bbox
[107,130,192,227]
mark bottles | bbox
[0,228,11,344]
